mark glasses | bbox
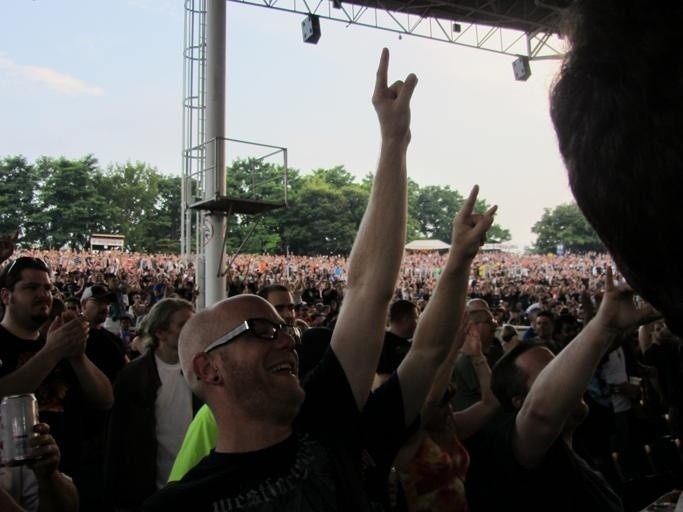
[194,316,304,380]
[7,257,45,276]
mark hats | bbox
[77,283,120,305]
[465,296,493,319]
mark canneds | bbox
[1,391,42,465]
